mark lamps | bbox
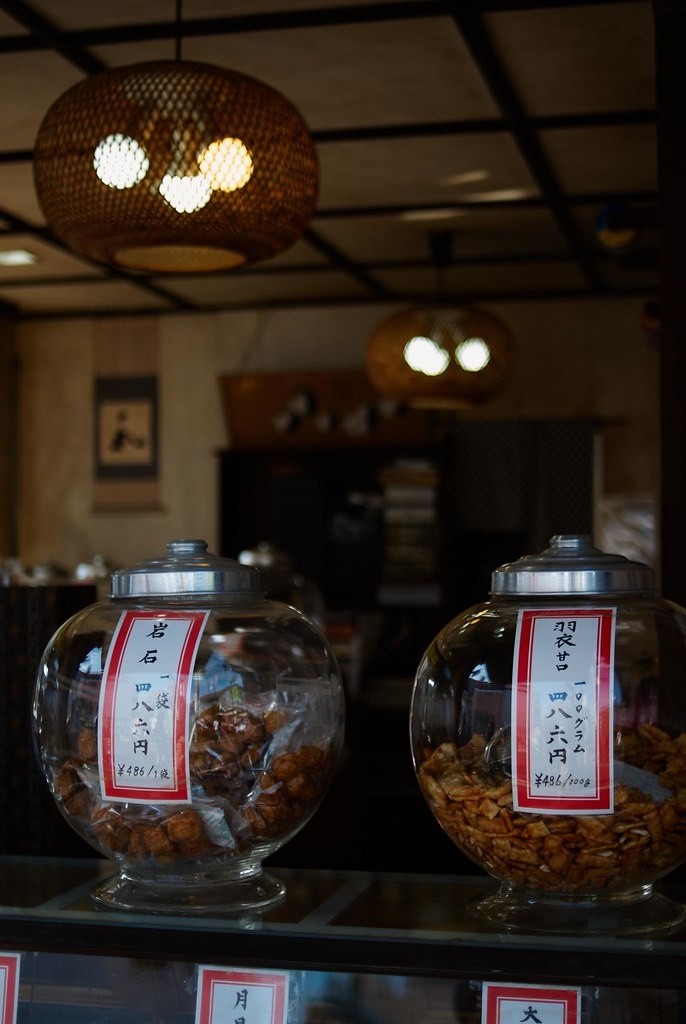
[363,230,514,412]
[30,0,319,279]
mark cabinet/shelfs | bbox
[209,414,632,712]
